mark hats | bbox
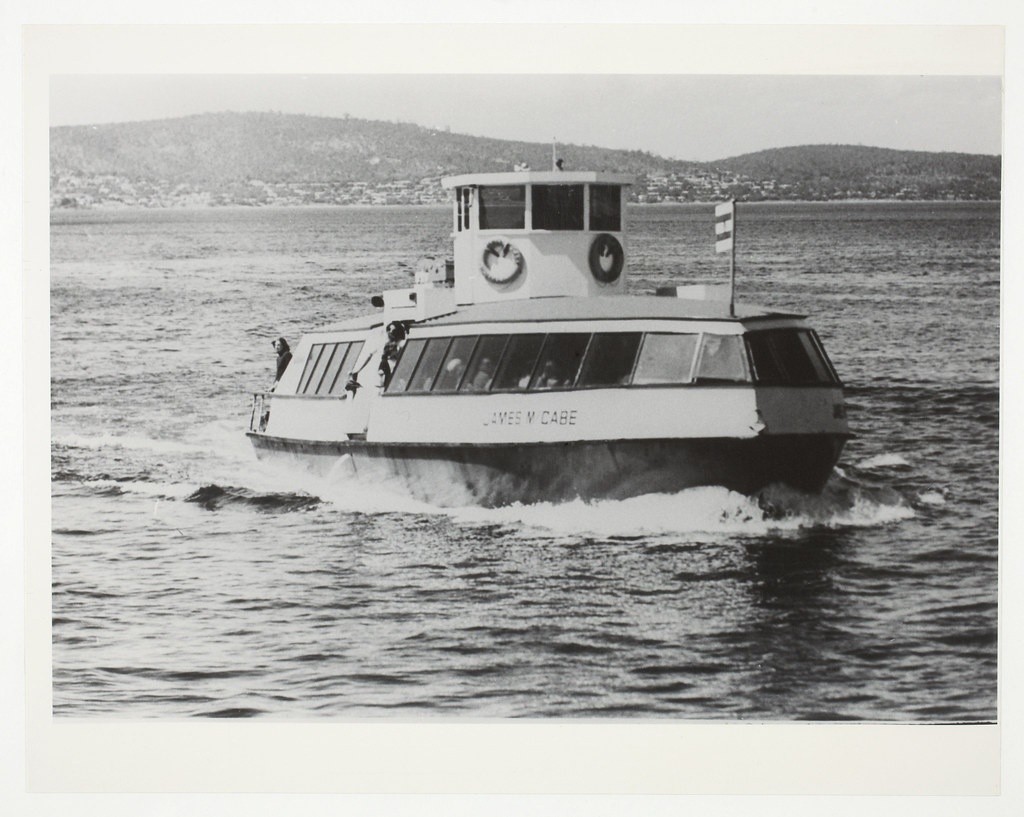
[446,358,461,372]
[386,322,405,340]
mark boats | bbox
[246,139,852,523]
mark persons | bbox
[442,357,471,395]
[472,357,493,393]
[270,337,293,393]
[517,360,573,392]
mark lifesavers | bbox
[478,239,524,283]
[589,233,625,284]
